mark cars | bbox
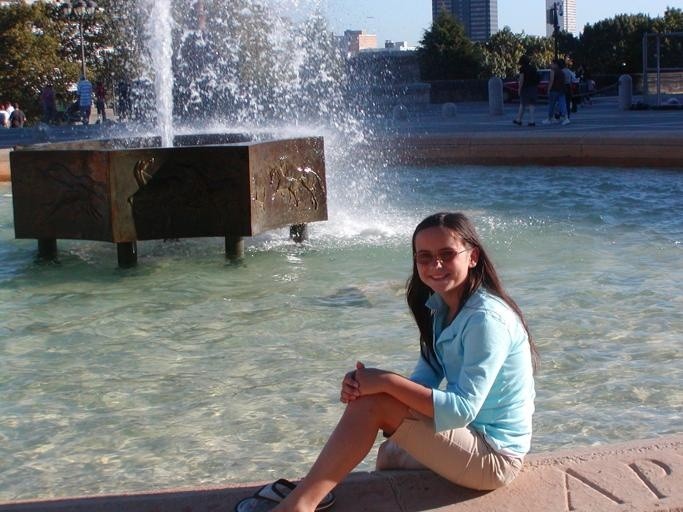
[500,66,596,106]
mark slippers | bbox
[232,477,338,511]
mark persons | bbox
[38,75,107,127]
[511,54,596,127]
[270,213,538,512]
[0,101,26,150]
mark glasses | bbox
[413,246,474,264]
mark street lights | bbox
[548,1,562,68]
[60,1,105,79]
